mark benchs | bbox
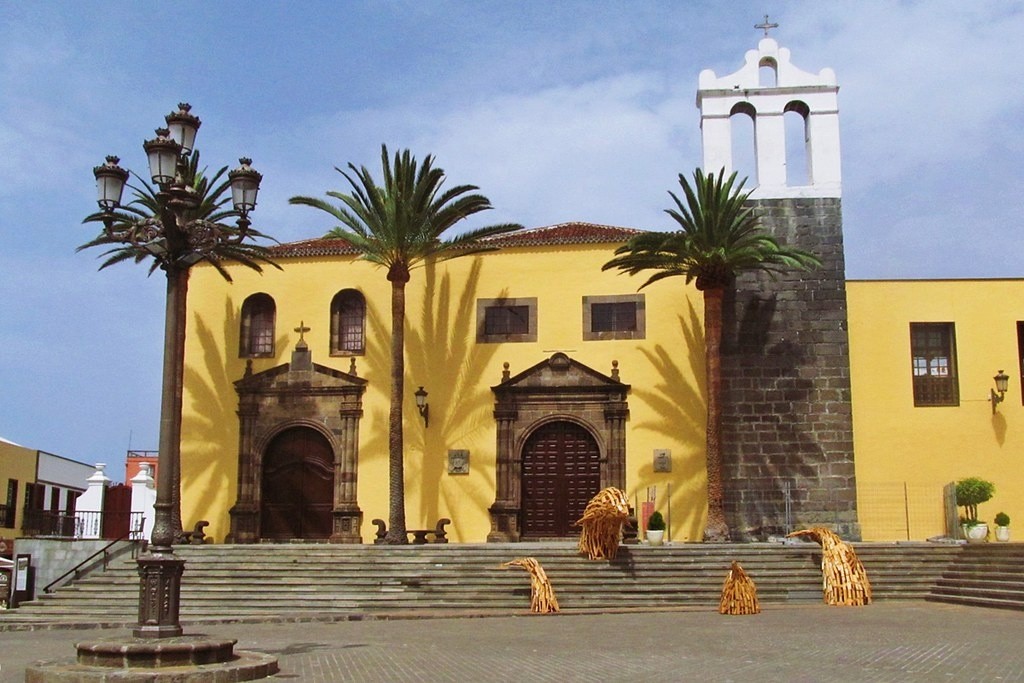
[372,518,451,545]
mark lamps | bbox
[413,386,429,429]
[990,369,1010,416]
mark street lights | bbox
[91,100,265,638]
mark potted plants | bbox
[955,476,997,543]
[645,511,667,546]
[993,511,1011,542]
